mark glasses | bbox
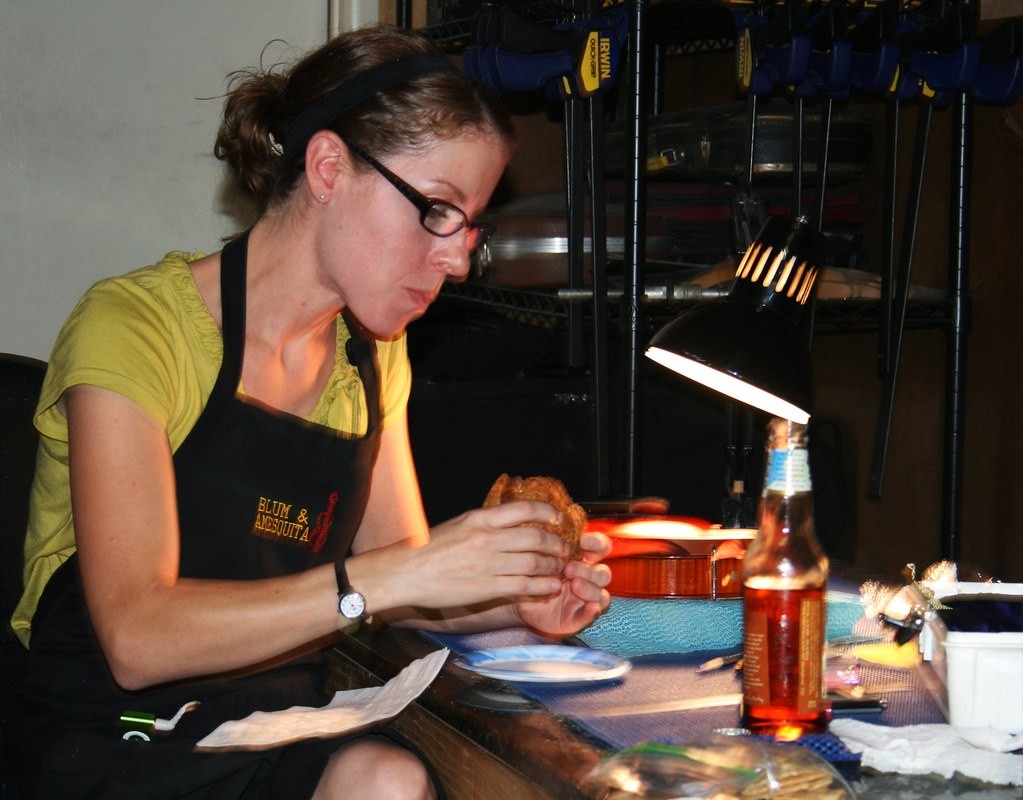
[339,135,498,260]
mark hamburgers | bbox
[482,472,586,583]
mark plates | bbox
[453,644,630,689]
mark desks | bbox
[314,570,1023,800]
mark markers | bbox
[826,701,887,719]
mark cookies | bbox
[741,754,846,800]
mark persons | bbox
[0,22,613,800]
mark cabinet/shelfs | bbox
[395,0,949,500]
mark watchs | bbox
[333,554,371,626]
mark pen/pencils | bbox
[696,651,744,673]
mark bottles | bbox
[743,416,835,742]
